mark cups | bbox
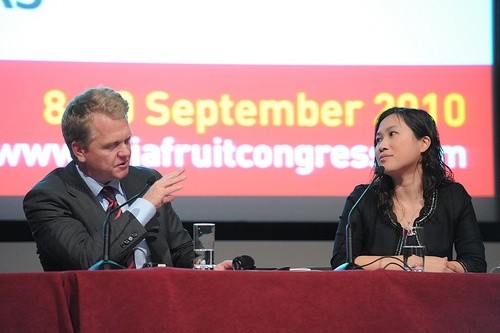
[193,223,215,270]
[403,227,426,272]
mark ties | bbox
[101,187,136,269]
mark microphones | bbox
[334,165,387,271]
[87,175,156,271]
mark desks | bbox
[0,267,500,333]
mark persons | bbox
[22,87,241,272]
[329,105,488,273]
